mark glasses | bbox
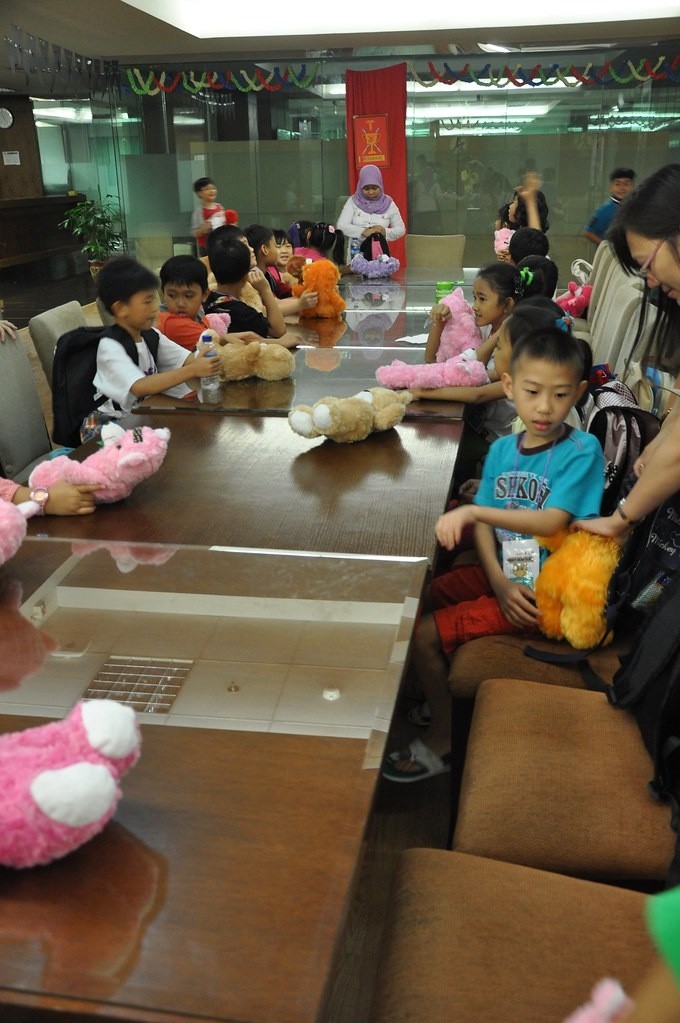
[199,186,216,193]
[639,232,667,283]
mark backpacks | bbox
[52,325,160,448]
[611,583,680,808]
[581,378,663,520]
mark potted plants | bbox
[57,194,129,281]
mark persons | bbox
[564,154,680,639]
[584,167,636,246]
[0,470,106,516]
[397,168,593,504]
[78,256,221,445]
[414,152,562,222]
[155,255,307,353]
[335,165,406,269]
[192,177,226,256]
[205,221,344,339]
[381,329,606,784]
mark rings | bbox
[639,464,645,473]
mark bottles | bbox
[198,334,222,390]
[434,282,455,306]
[351,237,361,260]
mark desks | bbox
[0,266,495,1023]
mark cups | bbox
[463,267,480,284]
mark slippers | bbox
[380,738,450,781]
[407,699,434,725]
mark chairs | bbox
[0,329,57,484]
[95,288,162,328]
[446,633,678,878]
[404,234,467,266]
[378,850,657,1022]
[438,197,468,235]
[570,237,658,382]
[28,300,88,388]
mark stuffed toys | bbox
[373,348,491,391]
[555,281,593,317]
[223,208,239,225]
[290,257,346,319]
[286,252,309,285]
[433,286,481,362]
[288,387,412,443]
[0,697,142,867]
[206,312,231,336]
[535,527,620,649]
[350,253,401,278]
[0,496,28,564]
[28,421,172,502]
[196,329,296,381]
[493,229,516,256]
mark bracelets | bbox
[616,503,641,525]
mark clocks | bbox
[0,107,14,128]
